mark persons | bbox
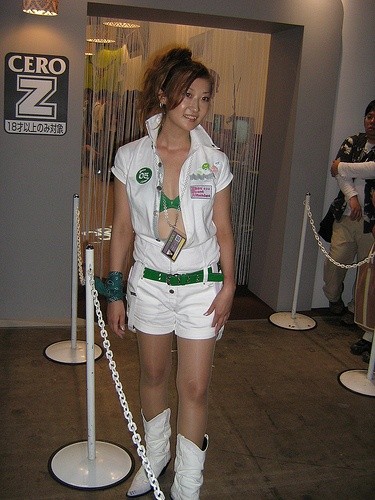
[351,192,375,363]
[319,100,375,327]
[81,88,148,183]
[105,48,235,500]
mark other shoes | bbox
[350,338,372,363]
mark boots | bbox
[125,408,172,497]
[171,433,209,500]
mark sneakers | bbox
[339,310,355,326]
[328,281,345,314]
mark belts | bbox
[144,265,225,287]
